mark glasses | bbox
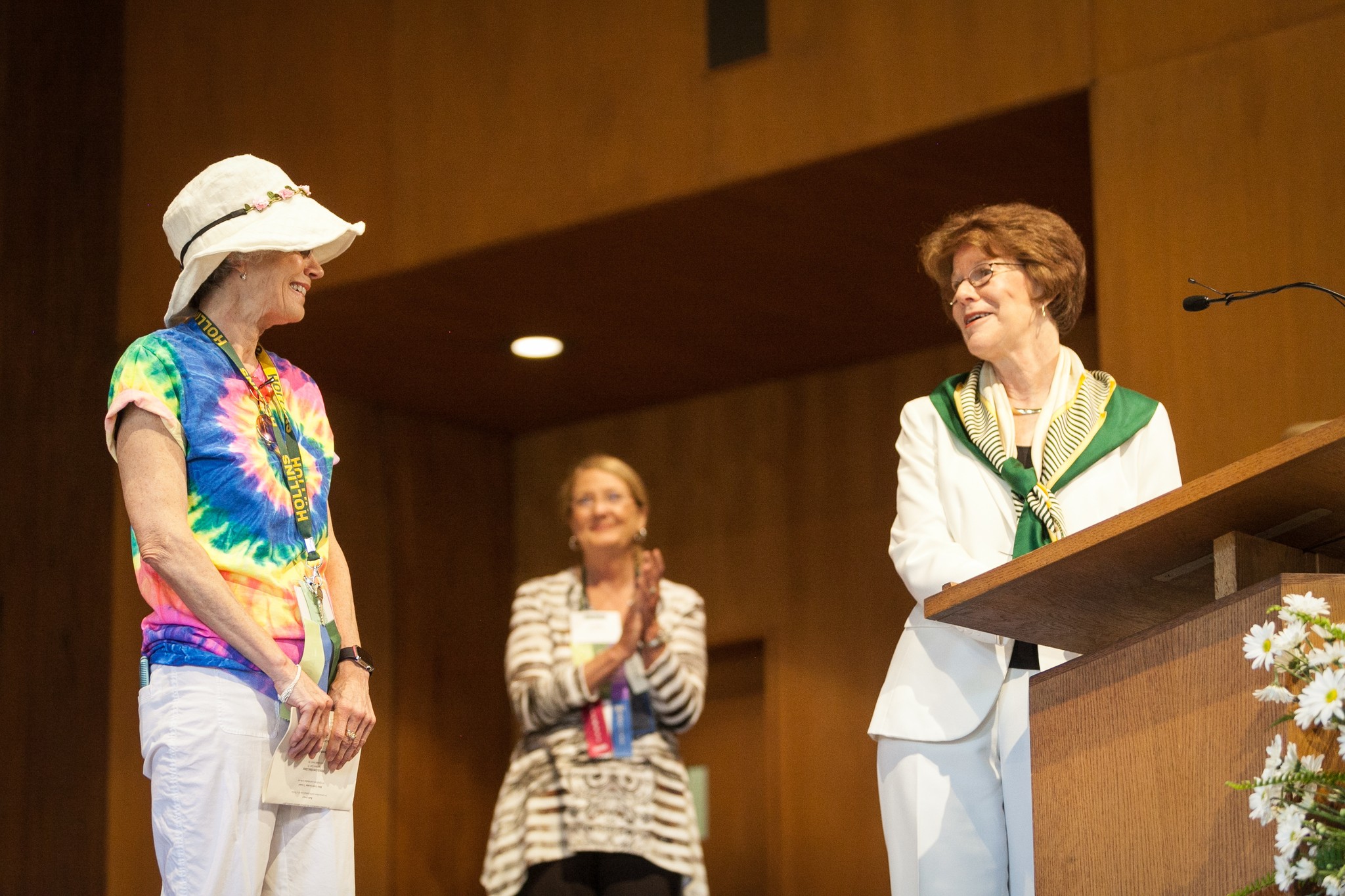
[943,262,1032,306]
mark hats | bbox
[163,154,367,328]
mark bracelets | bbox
[275,663,305,706]
[635,628,669,650]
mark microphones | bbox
[1182,278,1345,312]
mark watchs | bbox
[339,643,374,677]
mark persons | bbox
[868,205,1180,896]
[478,454,711,896]
[93,153,379,894]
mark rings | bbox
[342,729,357,740]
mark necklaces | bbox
[1009,403,1043,417]
